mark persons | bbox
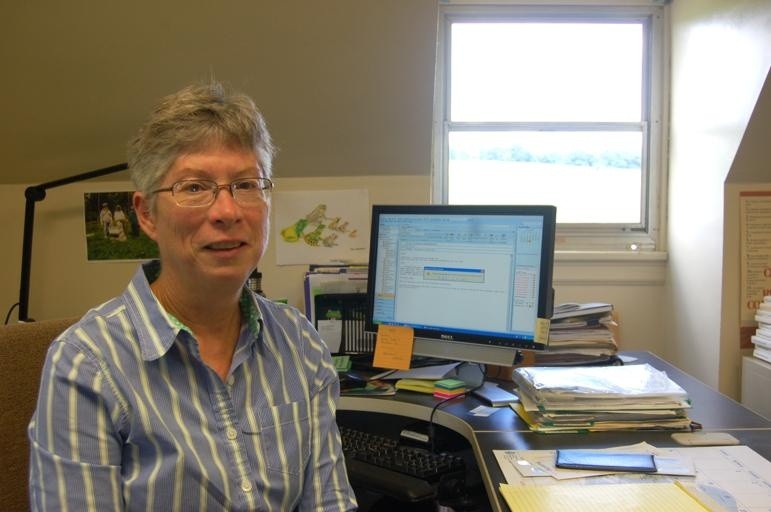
[113,204,126,229]
[129,205,140,235]
[99,201,111,237]
[105,219,127,240]
[24,77,360,512]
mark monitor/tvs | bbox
[364,203,558,407]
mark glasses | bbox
[151,178,273,208]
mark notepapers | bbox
[432,378,465,399]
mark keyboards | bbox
[335,427,468,502]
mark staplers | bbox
[337,371,367,390]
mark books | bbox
[751,295,771,367]
[475,383,518,409]
[505,363,696,432]
[553,446,660,473]
[535,299,621,367]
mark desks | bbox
[339,349,771,512]
[741,355,771,422]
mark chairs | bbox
[0,316,81,512]
